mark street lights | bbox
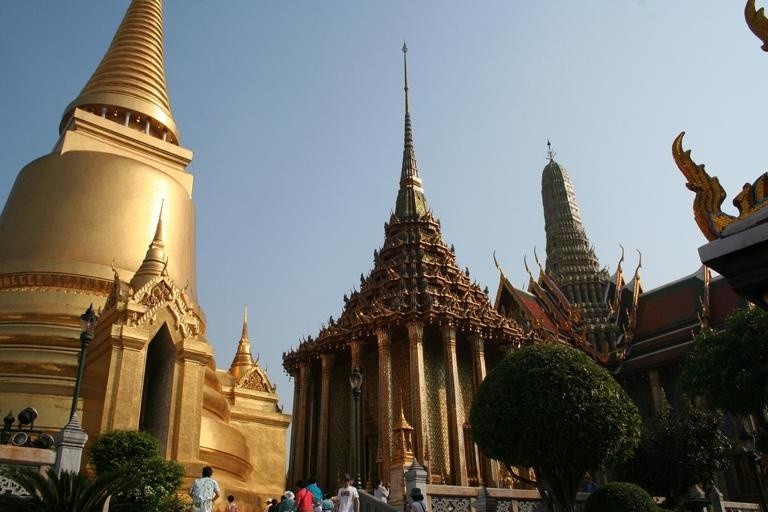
[67,300,102,425]
[348,362,366,490]
[737,422,767,512]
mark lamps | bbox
[33,433,55,449]
[17,406,39,431]
[12,431,32,449]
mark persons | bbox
[373,479,390,503]
[582,475,598,493]
[223,495,238,512]
[409,487,427,512]
[264,474,360,512]
[188,466,220,512]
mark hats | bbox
[410,488,422,496]
[285,491,295,499]
[343,474,350,480]
